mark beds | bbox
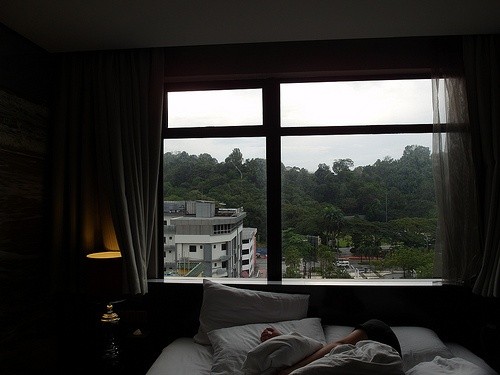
[146,336,496,375]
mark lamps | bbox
[86,250,123,323]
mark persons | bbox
[261,319,403,374]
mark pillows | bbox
[193,279,456,375]
